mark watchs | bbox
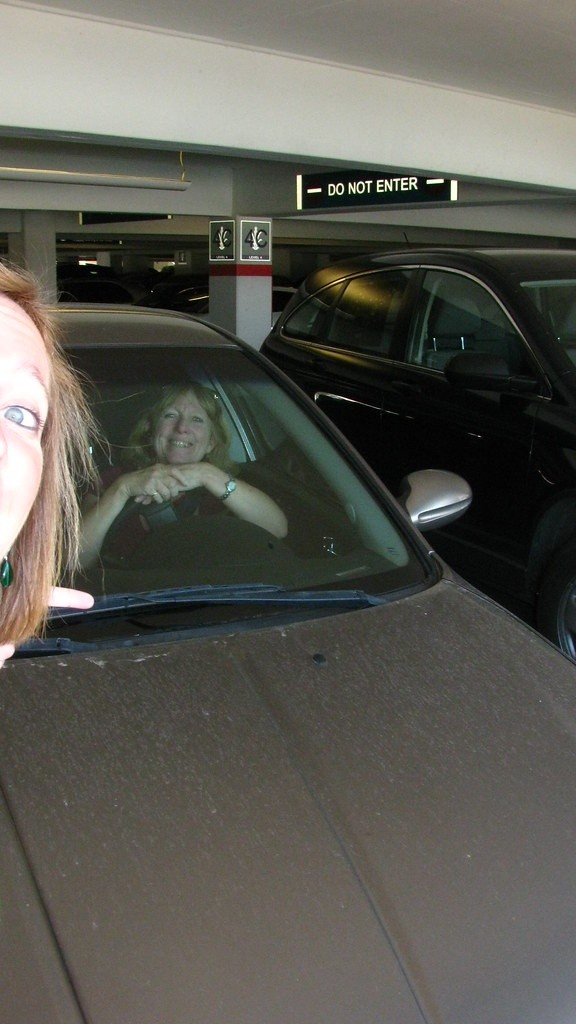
[214,474,237,502]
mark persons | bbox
[57,380,290,571]
[0,262,95,670]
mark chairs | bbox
[428,293,508,374]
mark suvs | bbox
[261,246,576,661]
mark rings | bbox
[151,491,158,497]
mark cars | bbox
[0,297,576,1024]
[57,262,319,336]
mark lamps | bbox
[0,148,195,194]
